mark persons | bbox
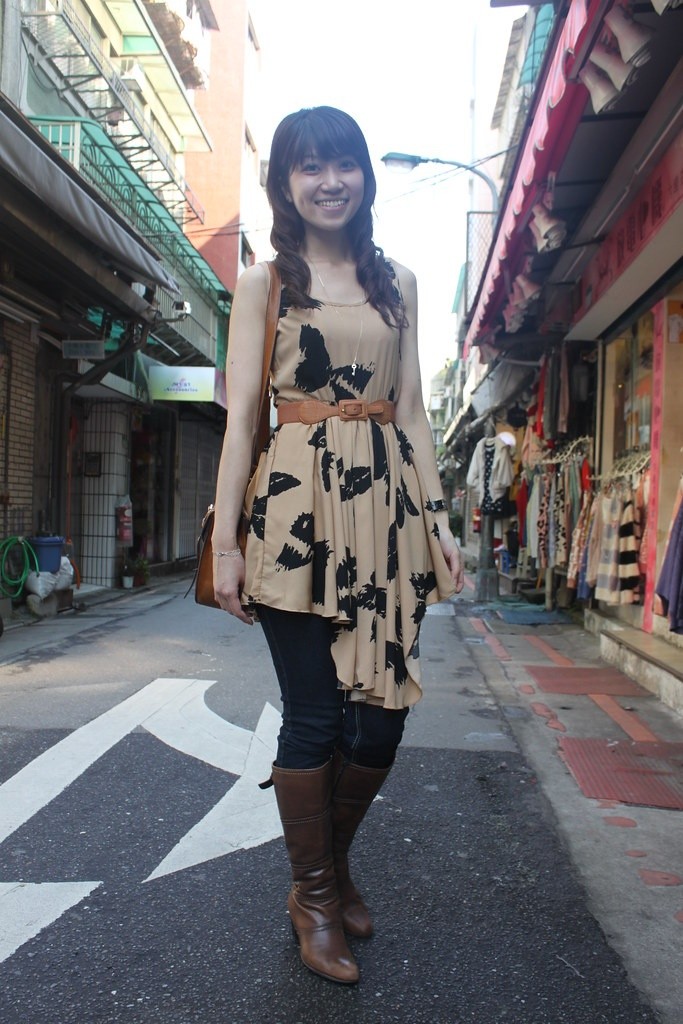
[208,108,459,980]
[505,521,519,567]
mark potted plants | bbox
[123,554,149,588]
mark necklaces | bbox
[305,251,367,376]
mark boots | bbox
[333,748,397,940]
[257,756,361,985]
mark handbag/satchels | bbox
[183,503,248,610]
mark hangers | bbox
[484,427,493,444]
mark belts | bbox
[277,398,398,425]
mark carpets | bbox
[558,735,683,808]
[500,605,573,623]
[523,665,656,696]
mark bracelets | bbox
[213,549,241,558]
[430,499,449,512]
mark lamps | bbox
[502,272,542,334]
[651,0,683,15]
[529,203,567,254]
[578,6,657,115]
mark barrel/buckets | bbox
[28,536,64,573]
